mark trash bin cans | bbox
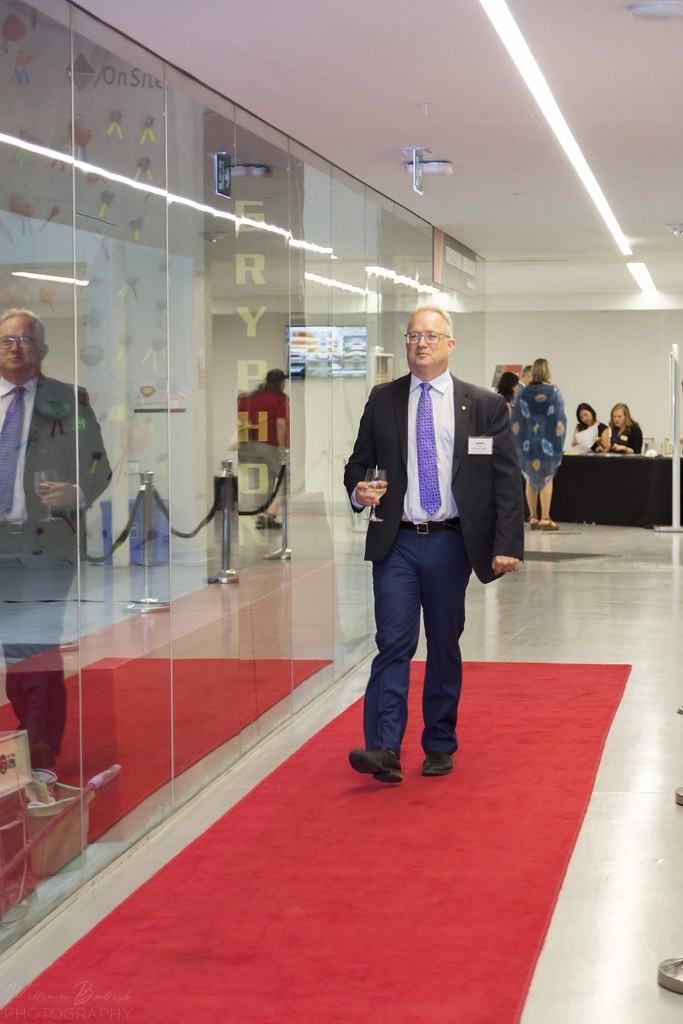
[128,499,168,568]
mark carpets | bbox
[0,658,334,925]
[0,658,635,1024]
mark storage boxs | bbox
[25,783,96,880]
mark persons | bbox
[238,368,288,529]
[0,307,113,770]
[572,402,609,453]
[343,304,524,785]
[490,359,567,531]
[596,403,643,454]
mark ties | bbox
[417,383,442,514]
[2,386,26,512]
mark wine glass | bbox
[364,468,387,522]
[34,470,58,520]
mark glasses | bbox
[406,330,452,346]
[0,336,36,348]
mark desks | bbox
[521,454,683,529]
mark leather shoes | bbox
[422,754,453,775]
[28,742,57,768]
[350,749,402,783]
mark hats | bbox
[266,369,288,383]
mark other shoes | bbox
[257,518,284,530]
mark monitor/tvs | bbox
[285,326,367,379]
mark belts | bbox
[0,522,23,536]
[399,520,458,534]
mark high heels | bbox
[540,519,560,530]
[529,516,541,530]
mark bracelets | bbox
[625,446,628,453]
